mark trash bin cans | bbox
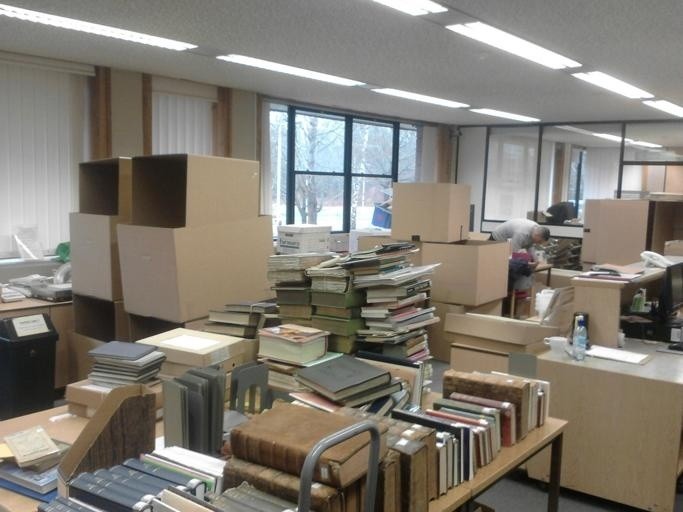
[0,313,58,422]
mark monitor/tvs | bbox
[658,262,683,325]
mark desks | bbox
[528,335,683,511]
[1,391,569,512]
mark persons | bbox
[487,217,551,255]
[543,197,577,226]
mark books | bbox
[574,270,642,282]
[590,262,645,275]
[1,239,551,512]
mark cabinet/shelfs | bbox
[1,304,78,389]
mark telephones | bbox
[640,249,671,269]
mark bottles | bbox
[572,319,587,361]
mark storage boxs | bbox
[276,223,332,256]
[356,182,561,373]
[68,153,133,304]
[115,155,273,326]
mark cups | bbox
[617,332,625,348]
[544,336,567,354]
